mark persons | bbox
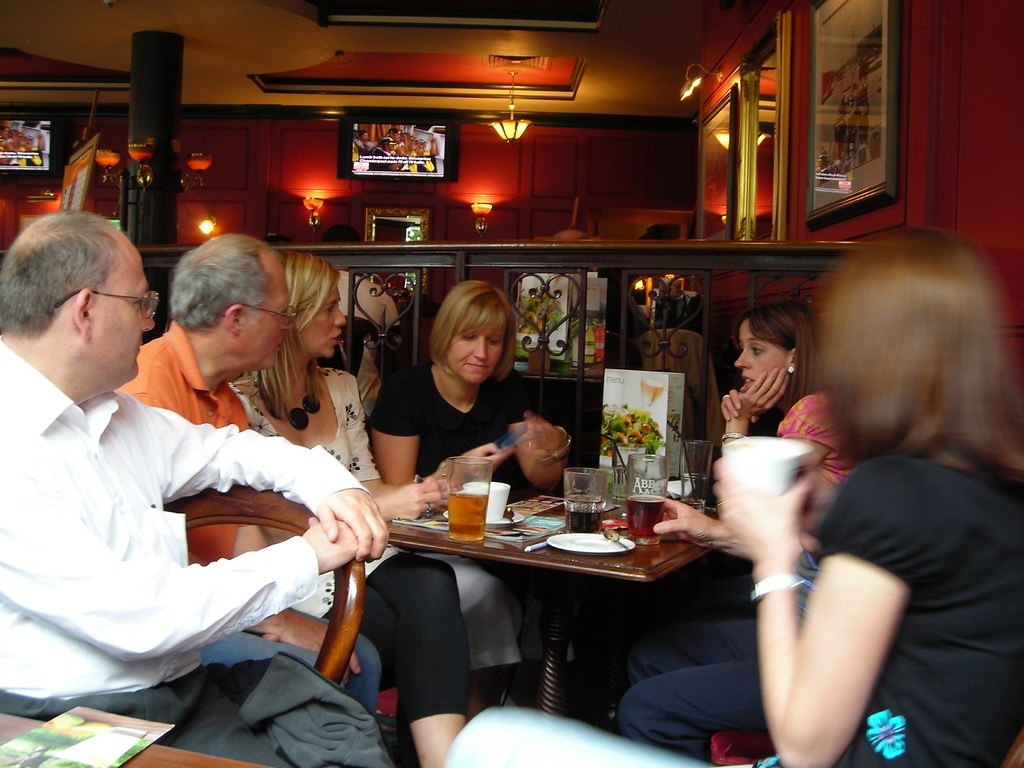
[0,125,44,166]
[226,252,472,768]
[370,280,572,722]
[0,210,394,768]
[114,235,382,716]
[617,298,849,764]
[833,82,871,163]
[714,228,1024,768]
[354,128,439,173]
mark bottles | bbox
[595,315,605,362]
[572,333,579,366]
[585,318,594,364]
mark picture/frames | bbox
[805,0,905,232]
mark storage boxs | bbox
[599,369,685,498]
[514,271,599,362]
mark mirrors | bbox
[696,81,738,242]
[736,8,793,245]
[365,207,432,305]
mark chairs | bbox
[637,328,727,447]
[164,484,366,689]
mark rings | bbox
[423,503,432,520]
[529,441,532,450]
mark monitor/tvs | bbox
[337,115,461,182]
[0,115,64,178]
[812,140,855,194]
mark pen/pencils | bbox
[524,541,549,552]
[411,473,422,481]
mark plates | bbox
[443,511,524,529]
[547,533,636,556]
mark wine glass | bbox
[641,375,666,413]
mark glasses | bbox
[53,288,159,320]
[221,302,296,325]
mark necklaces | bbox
[253,362,320,430]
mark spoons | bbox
[604,529,630,553]
[503,507,516,524]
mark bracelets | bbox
[752,573,803,605]
[533,426,572,463]
[722,432,746,443]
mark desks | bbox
[0,712,272,768]
[382,497,721,719]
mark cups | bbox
[612,443,648,508]
[54,726,149,768]
[564,468,608,533]
[628,454,669,545]
[462,482,511,521]
[447,457,493,542]
[723,436,814,499]
[680,441,713,513]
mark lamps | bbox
[489,72,534,148]
[26,188,58,203]
[178,152,214,201]
[471,202,492,236]
[758,127,773,147]
[713,126,730,150]
[678,63,722,100]
[303,198,323,231]
[127,142,155,194]
[95,149,122,187]
[199,210,217,235]
